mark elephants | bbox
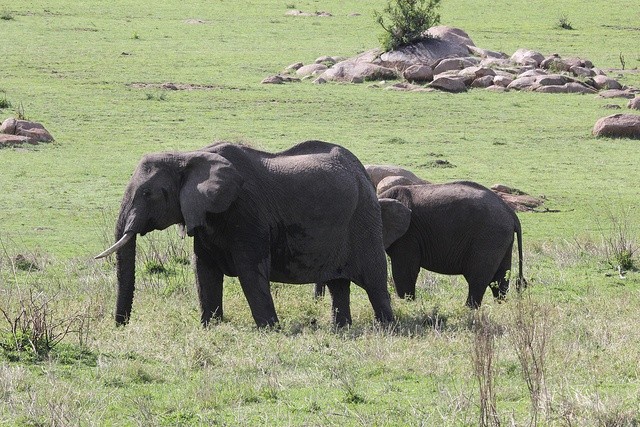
[93,138,396,334]
[311,180,528,312]
